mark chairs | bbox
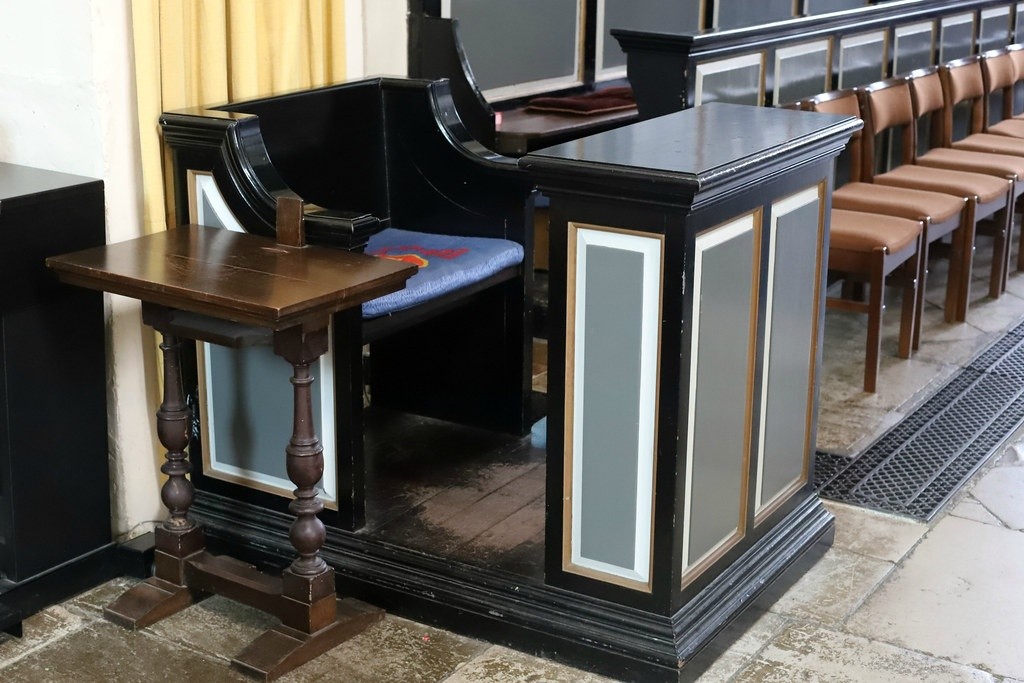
[160,78,537,532]
[787,41,1024,394]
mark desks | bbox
[517,102,864,619]
[44,223,418,683]
[493,104,641,154]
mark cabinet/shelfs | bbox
[0,160,123,639]
